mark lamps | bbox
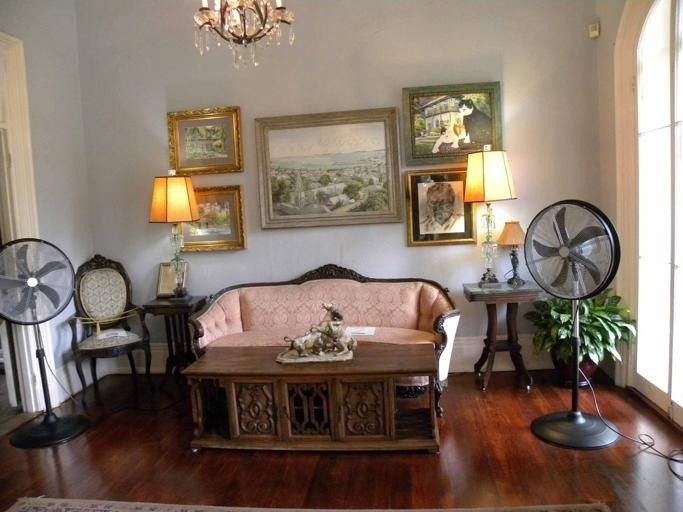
[463,146,518,287]
[192,0,296,69]
[149,169,200,302]
[497,222,527,285]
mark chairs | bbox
[67,254,152,410]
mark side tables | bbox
[142,297,219,399]
[463,282,548,392]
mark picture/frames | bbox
[156,262,187,297]
[404,169,478,246]
[255,106,404,228]
[175,184,245,253]
[401,80,504,166]
[166,106,244,175]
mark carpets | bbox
[5,496,611,512]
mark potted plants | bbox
[522,282,636,388]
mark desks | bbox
[181,343,440,457]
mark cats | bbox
[431,120,471,154]
[458,99,492,144]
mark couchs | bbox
[188,265,461,418]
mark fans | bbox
[524,199,619,450]
[0,238,90,449]
